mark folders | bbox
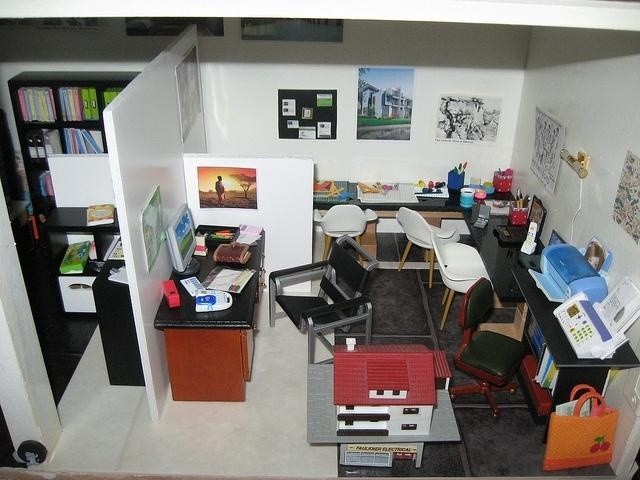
[20,121,46,161]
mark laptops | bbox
[494,194,547,244]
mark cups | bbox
[509,201,528,225]
[459,187,476,208]
[493,172,512,193]
[447,171,465,190]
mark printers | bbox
[552,275,640,359]
[528,244,608,305]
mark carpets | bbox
[332,265,622,479]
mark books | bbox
[87,204,115,227]
[18,86,120,196]
[527,313,559,396]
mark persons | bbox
[584,242,603,273]
[215,176,225,207]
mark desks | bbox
[314,181,465,262]
[459,184,546,343]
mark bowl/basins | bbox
[475,181,494,199]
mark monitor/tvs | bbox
[166,204,201,276]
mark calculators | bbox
[179,276,206,297]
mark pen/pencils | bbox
[516,188,530,208]
[227,269,254,291]
[498,168,502,174]
[210,230,235,240]
[454,162,467,174]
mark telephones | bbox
[521,222,538,255]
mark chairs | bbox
[429,234,495,331]
[444,275,528,422]
[267,233,381,364]
[316,204,379,270]
[390,207,461,291]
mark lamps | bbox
[560,142,592,181]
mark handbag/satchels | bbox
[541,382,619,472]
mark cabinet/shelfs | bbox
[508,264,640,445]
[152,224,266,403]
[46,204,147,386]
[7,70,144,224]
